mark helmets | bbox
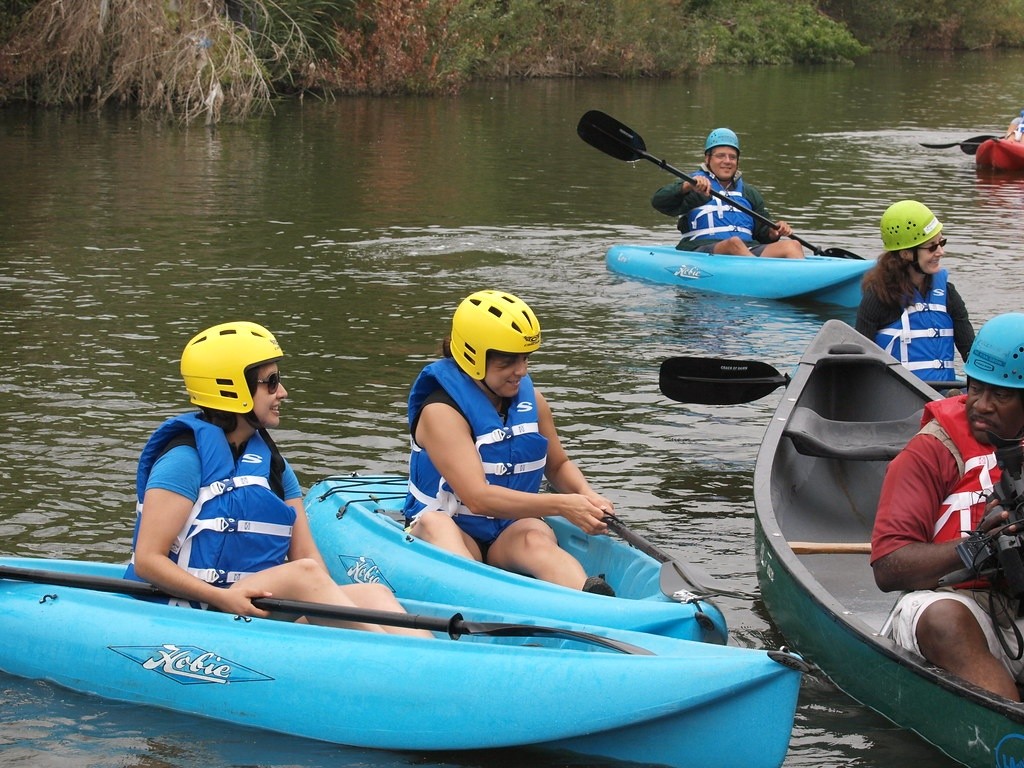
[962,313,1024,388]
[704,128,740,153]
[450,290,540,380]
[180,321,283,414]
[880,200,943,250]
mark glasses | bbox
[710,153,738,159]
[246,371,281,395]
[912,237,947,252]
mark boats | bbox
[754,317,1024,768]
[304,471,728,648]
[975,135,1024,172]
[0,554,809,768]
[604,242,878,309]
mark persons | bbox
[855,199,975,398]
[122,321,435,638]
[1004,110,1024,142]
[870,312,1024,702]
[401,290,617,596]
[651,128,806,257]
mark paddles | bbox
[918,141,982,149]
[656,354,967,406]
[961,135,1005,155]
[575,108,868,260]
[1,565,656,657]
[544,482,745,604]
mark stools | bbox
[786,410,927,462]
